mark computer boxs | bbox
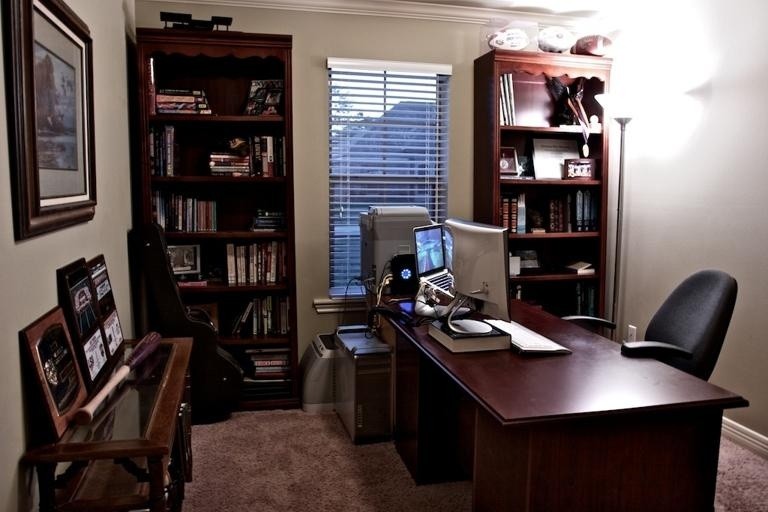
[360,206,438,295]
[334,326,393,446]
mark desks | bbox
[372,300,748,509]
[23,329,195,512]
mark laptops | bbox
[413,223,455,299]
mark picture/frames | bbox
[185,301,219,336]
[498,145,519,175]
[163,243,201,276]
[9,2,100,243]
[19,309,89,443]
[561,158,596,181]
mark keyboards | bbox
[483,319,573,356]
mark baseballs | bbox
[589,114,600,123]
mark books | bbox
[499,73,599,316]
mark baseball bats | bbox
[71,332,161,425]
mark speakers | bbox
[390,254,419,298]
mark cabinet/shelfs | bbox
[133,28,302,410]
[473,52,613,338]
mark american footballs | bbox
[537,26,576,53]
[570,35,613,56]
[488,28,530,51]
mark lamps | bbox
[592,89,655,344]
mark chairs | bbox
[557,265,740,382]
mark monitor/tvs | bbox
[444,216,511,335]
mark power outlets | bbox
[626,321,637,344]
